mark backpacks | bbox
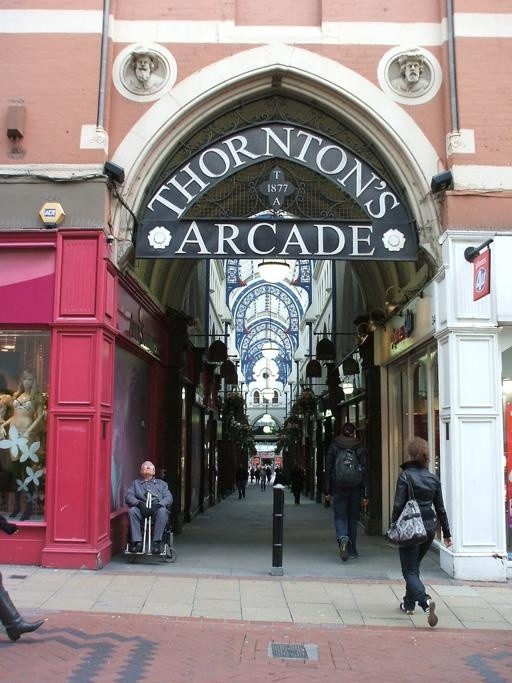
[332,448,362,487]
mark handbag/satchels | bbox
[385,499,428,546]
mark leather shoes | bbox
[130,542,142,552]
[152,542,160,553]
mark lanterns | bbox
[275,460,282,465]
[255,461,260,464]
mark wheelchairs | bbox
[124,505,180,566]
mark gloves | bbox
[137,502,148,517]
[148,504,160,515]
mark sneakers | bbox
[425,599,438,626]
[400,603,414,615]
[340,538,349,561]
[349,554,357,558]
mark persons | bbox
[2,368,46,522]
[250,463,287,492]
[1,368,45,641]
[386,435,454,627]
[288,462,304,505]
[323,422,370,562]
[124,458,173,555]
[0,392,16,505]
[234,463,249,500]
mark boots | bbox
[0,592,44,642]
